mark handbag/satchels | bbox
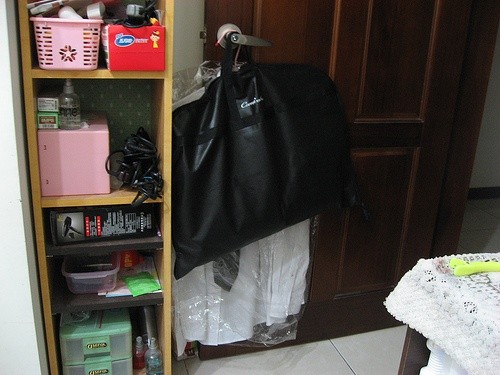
[171,29,353,280]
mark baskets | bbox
[30,15,104,71]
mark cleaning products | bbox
[59,79,81,131]
[133,336,146,369]
[145,337,162,375]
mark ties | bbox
[212,248,242,292]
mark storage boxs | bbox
[62,355,134,375]
[37,114,59,129]
[29,15,104,70]
[37,96,61,112]
[59,309,132,366]
[48,208,156,244]
[61,255,120,294]
[38,111,111,196]
[100,19,165,72]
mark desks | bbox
[382,252,500,375]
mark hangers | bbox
[215,29,245,74]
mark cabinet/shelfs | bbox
[16,0,172,375]
